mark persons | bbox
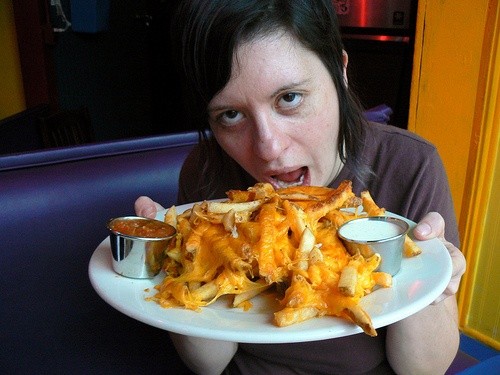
[135,0,466,375]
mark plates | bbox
[88,197,453,344]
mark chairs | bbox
[38,107,96,148]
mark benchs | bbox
[1,105,393,375]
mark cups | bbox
[337,216,409,279]
[105,217,176,280]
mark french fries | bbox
[164,181,423,337]
